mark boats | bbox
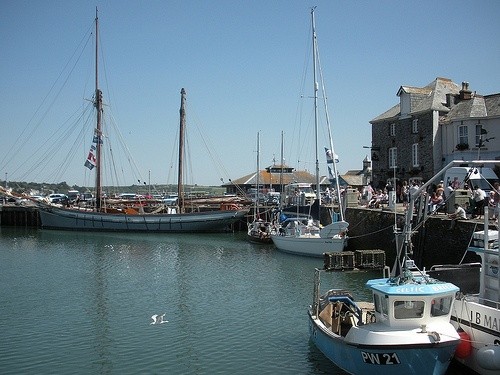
[306,210,462,375]
[390,158,500,375]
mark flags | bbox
[84,132,105,171]
[324,147,339,182]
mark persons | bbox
[368,177,500,232]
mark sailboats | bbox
[245,129,287,245]
[0,5,254,233]
[267,4,350,256]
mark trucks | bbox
[285,183,317,205]
[442,166,499,206]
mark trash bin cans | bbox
[447,189,471,214]
[344,189,358,207]
